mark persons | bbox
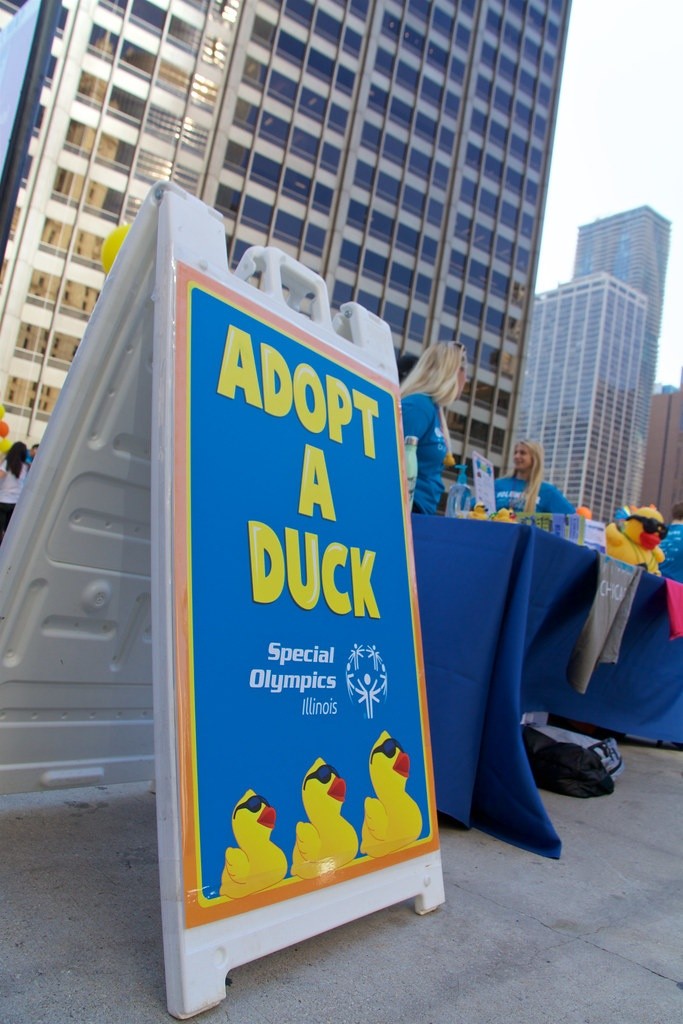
[400,341,468,514]
[0,442,39,544]
[671,502,683,525]
[494,440,576,514]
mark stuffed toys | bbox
[469,503,488,520]
[100,226,132,275]
[0,405,12,456]
[606,504,665,576]
[492,509,516,522]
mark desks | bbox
[411,510,683,860]
